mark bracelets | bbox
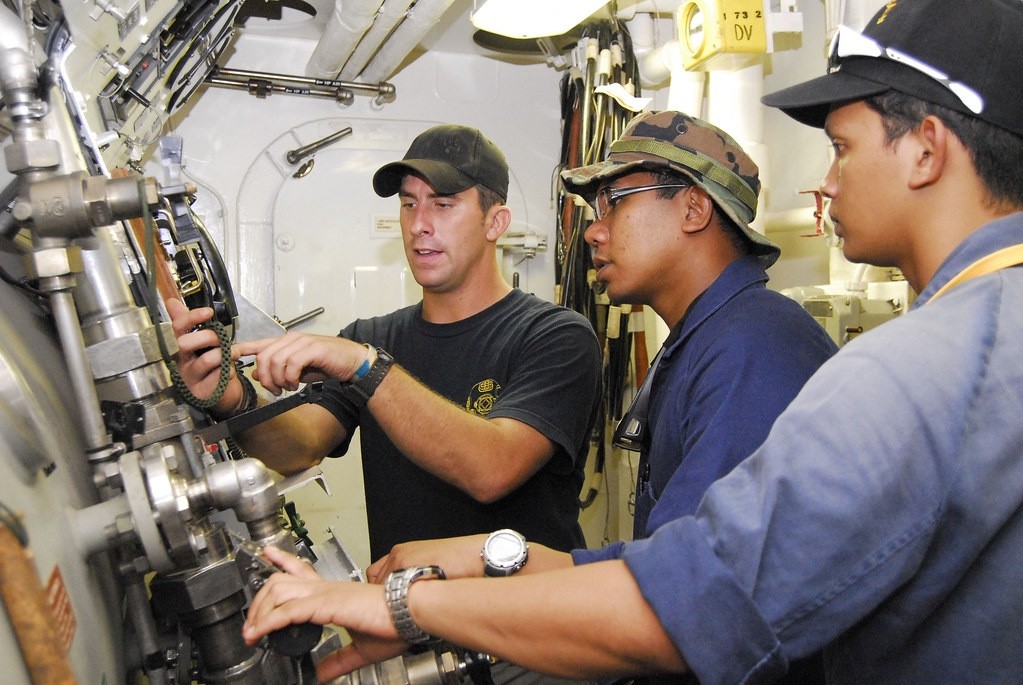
[347,341,378,383]
[203,373,259,422]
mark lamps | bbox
[467,0,613,40]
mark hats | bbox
[373,122,511,203]
[560,109,782,271]
[761,0,1023,136]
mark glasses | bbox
[825,22,984,115]
[594,183,689,222]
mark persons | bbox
[367,110,845,685]
[167,124,603,559]
[242,0,1023,685]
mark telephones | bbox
[173,209,239,326]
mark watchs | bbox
[382,564,448,647]
[340,347,396,407]
[479,527,529,578]
